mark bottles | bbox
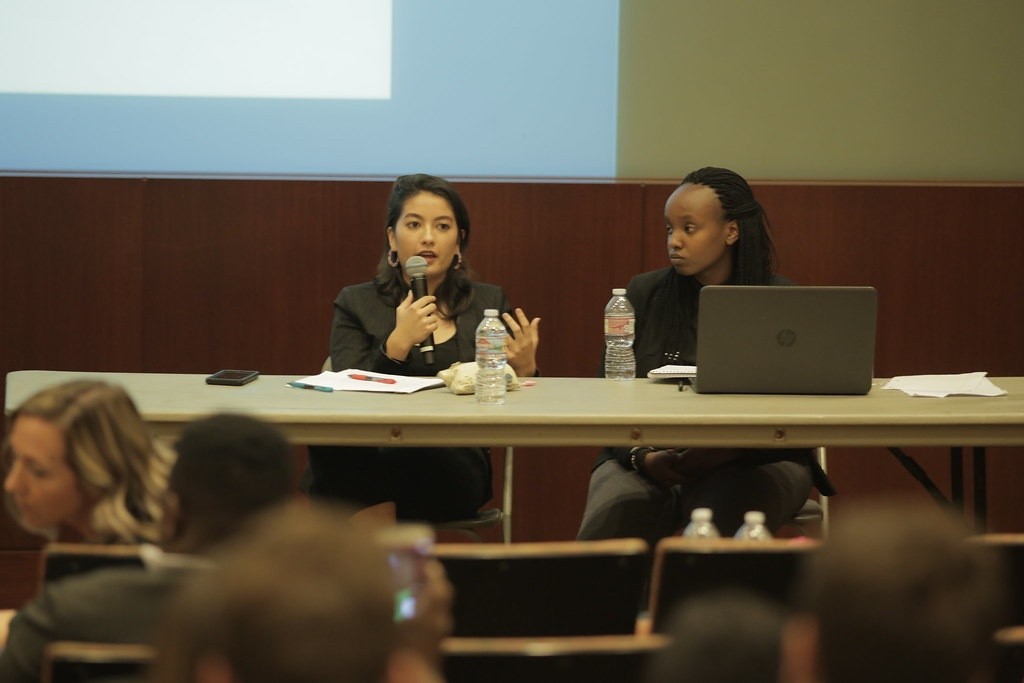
[604,289,635,380]
[682,508,721,539]
[475,309,507,405]
[733,511,774,540]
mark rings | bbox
[677,454,683,458]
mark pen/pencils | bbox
[286,382,333,392]
[519,381,537,387]
[678,377,683,391]
[347,374,397,384]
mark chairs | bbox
[41,532,1024,683]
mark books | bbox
[647,365,698,379]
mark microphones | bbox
[405,256,435,365]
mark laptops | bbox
[688,286,880,393]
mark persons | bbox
[0,379,453,683]
[304,174,540,536]
[653,498,1006,683]
[569,167,817,620]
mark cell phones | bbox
[381,541,430,621]
[206,370,260,387]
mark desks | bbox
[5,369,1024,544]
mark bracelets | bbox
[629,446,655,470]
